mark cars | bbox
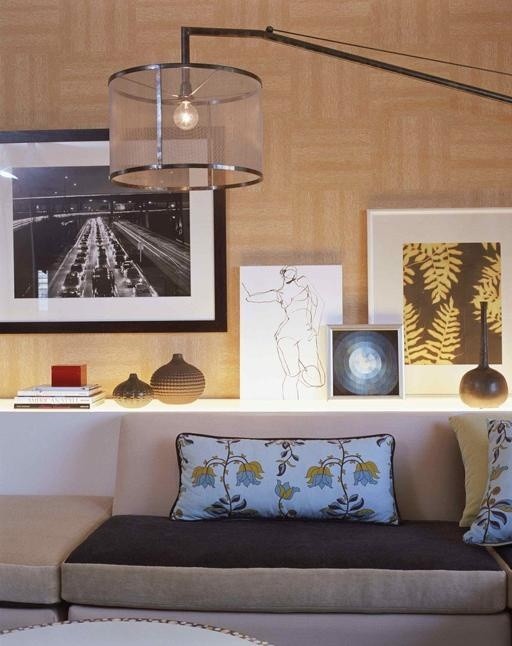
[60,219,152,298]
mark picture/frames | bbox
[366,207,512,396]
[0,131,227,334]
[326,325,405,401]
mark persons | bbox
[245,266,327,406]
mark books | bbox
[13,382,107,410]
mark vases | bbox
[150,354,206,404]
[113,373,154,409]
[459,301,510,408]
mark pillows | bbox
[461,415,512,548]
[168,433,400,525]
[448,412,512,527]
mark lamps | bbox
[106,26,512,191]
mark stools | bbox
[0,494,114,630]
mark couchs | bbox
[59,411,512,646]
